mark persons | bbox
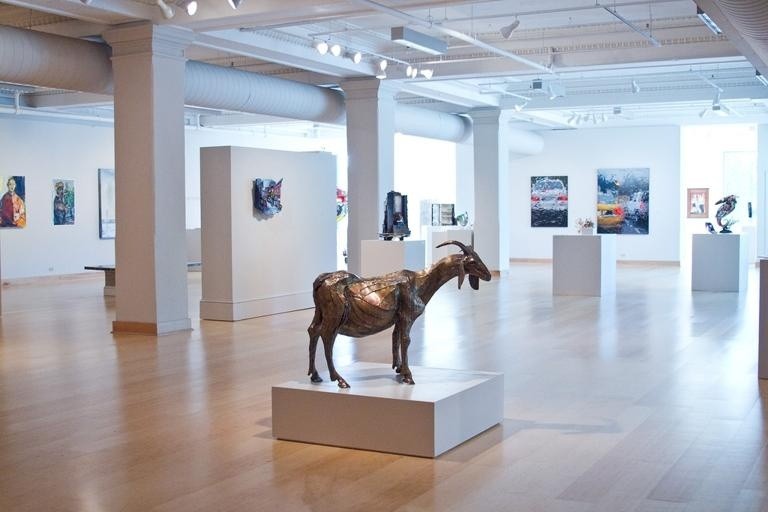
[53,181,68,225]
[0,178,26,227]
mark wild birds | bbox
[706,223,717,234]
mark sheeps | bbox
[308,232,491,388]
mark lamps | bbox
[412,68,418,79]
[499,19,522,41]
[161,0,198,23]
[396,63,411,76]
[310,38,330,57]
[371,57,387,70]
[346,51,363,64]
[563,111,608,127]
[630,80,640,94]
[697,107,710,118]
[328,43,341,59]
[513,101,528,111]
[547,85,556,100]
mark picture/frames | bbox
[687,188,709,218]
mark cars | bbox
[531,180,568,224]
[598,192,649,233]
